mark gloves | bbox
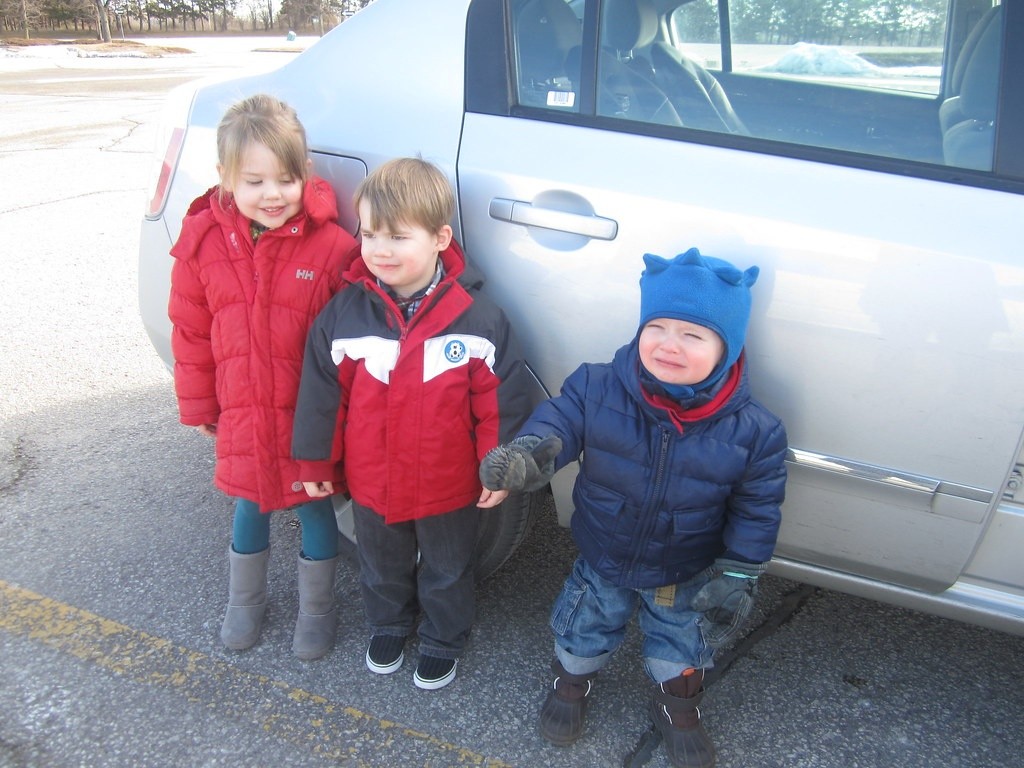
[479,435,563,494]
[689,559,768,648]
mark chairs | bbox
[606,1,744,141]
[517,0,685,117]
[940,0,1005,171]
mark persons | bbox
[480,248,788,768]
[292,159,527,689]
[169,97,361,659]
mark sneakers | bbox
[366,635,407,674]
[413,654,457,690]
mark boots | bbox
[292,549,339,660]
[648,667,715,768]
[221,542,271,650]
[537,651,600,747]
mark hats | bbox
[635,247,760,399]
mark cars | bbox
[137,1,1024,643]
